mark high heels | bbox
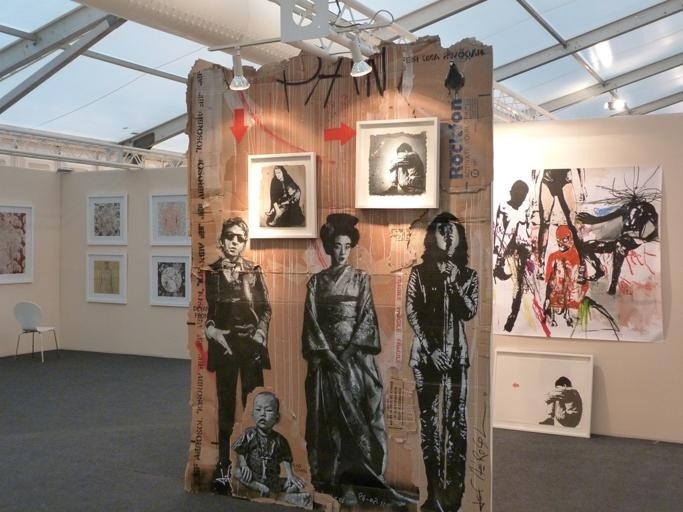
[503,312,518,333]
[492,267,513,285]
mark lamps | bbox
[604,94,626,112]
[204,19,394,93]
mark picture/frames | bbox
[353,115,441,212]
[148,192,191,247]
[245,150,319,241]
[491,347,594,440]
[148,254,193,308]
[0,201,35,285]
[84,250,128,306]
[85,192,129,247]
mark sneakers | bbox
[211,461,232,493]
[381,186,398,195]
[538,417,554,425]
[420,485,465,512]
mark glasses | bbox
[223,230,246,243]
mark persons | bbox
[301,213,406,511]
[379,142,425,195]
[494,169,588,337]
[405,210,480,512]
[539,376,583,433]
[265,166,305,227]
[204,217,272,487]
[232,391,311,508]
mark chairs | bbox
[11,300,62,366]
